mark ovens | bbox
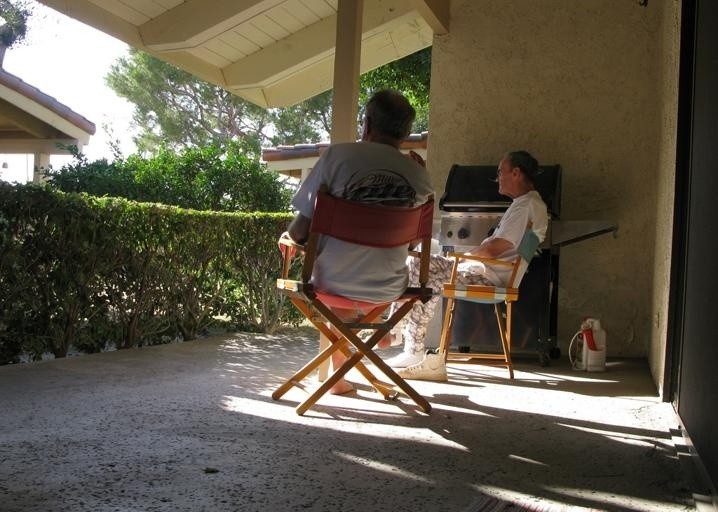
[439,163,563,220]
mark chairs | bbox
[443,218,543,381]
[272,190,439,417]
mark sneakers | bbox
[395,348,447,382]
[383,352,420,367]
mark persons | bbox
[378,147,549,369]
[272,87,437,397]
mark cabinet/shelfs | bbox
[440,246,562,366]
